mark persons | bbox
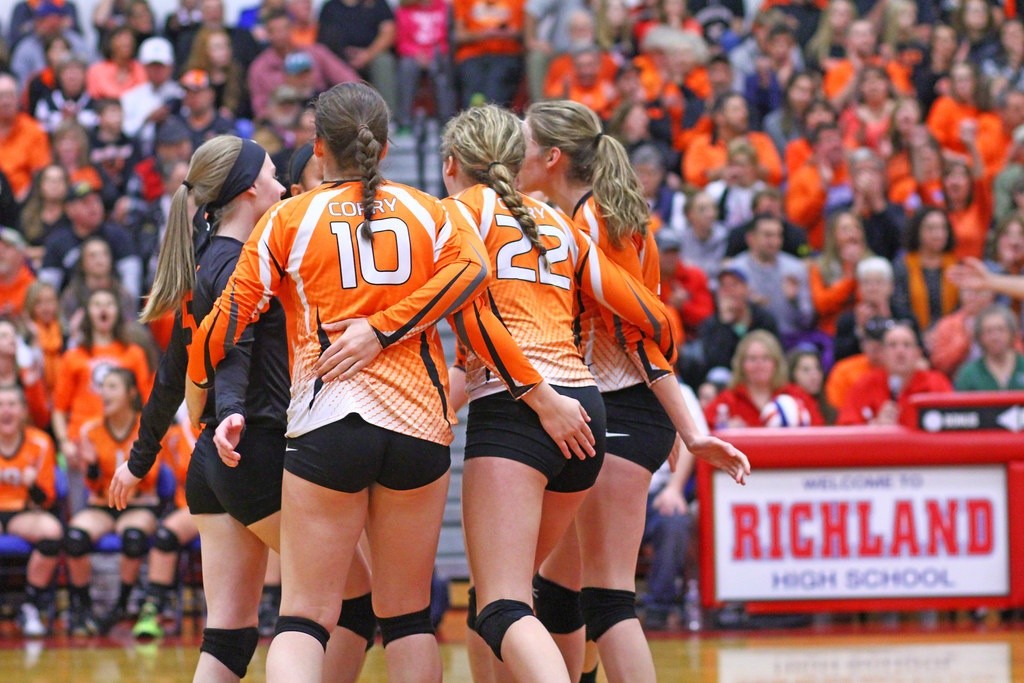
[0,0,1024,683]
[704,328,824,430]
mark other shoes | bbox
[73,594,91,637]
[20,603,47,638]
[130,600,165,638]
[645,611,669,630]
[85,609,127,635]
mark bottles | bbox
[685,579,702,633]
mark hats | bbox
[62,181,92,203]
[136,36,176,66]
[182,70,211,90]
[283,51,313,72]
[158,115,192,143]
